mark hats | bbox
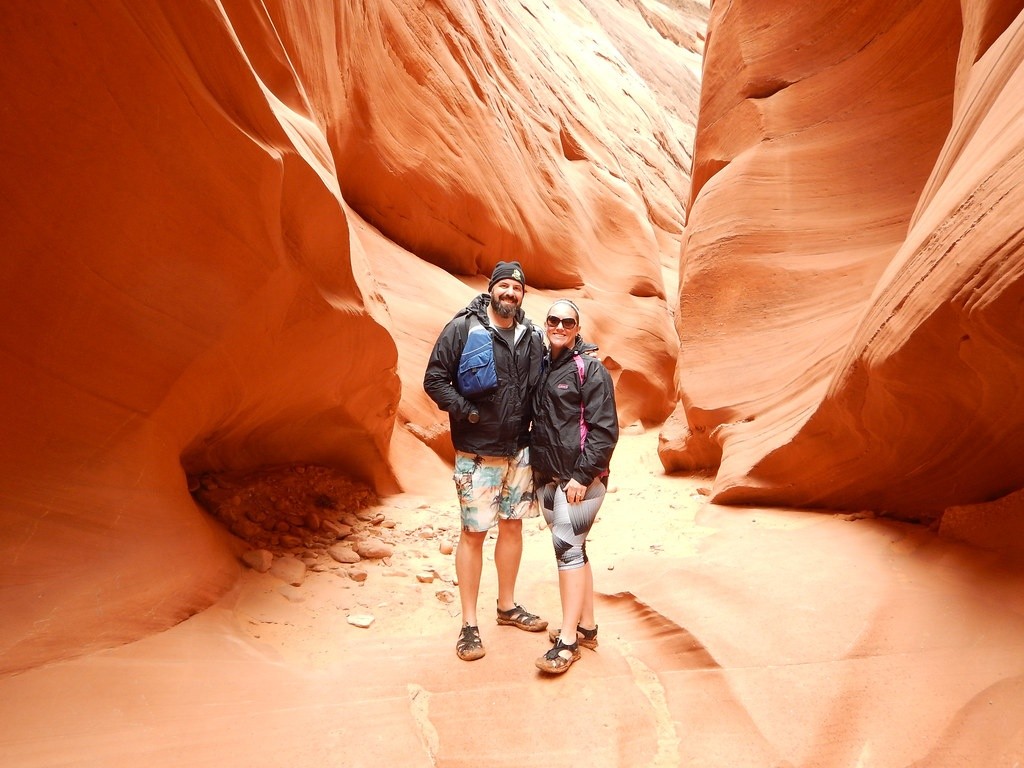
[488,260,524,296]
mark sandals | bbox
[535,633,583,675]
[550,625,599,651]
[457,624,485,661]
[495,599,548,631]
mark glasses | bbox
[547,315,578,329]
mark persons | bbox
[529,301,619,674]
[424,261,549,661]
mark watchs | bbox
[468,409,479,424]
